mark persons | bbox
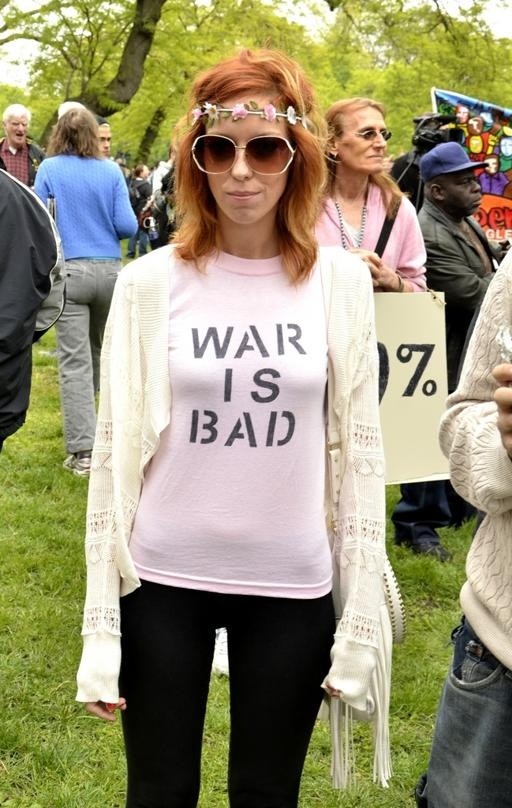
[0,101,47,188]
[476,153,511,198]
[310,95,428,292]
[73,47,408,808]
[32,100,138,479]
[123,163,153,260]
[92,110,112,161]
[0,166,68,460]
[147,141,177,203]
[412,243,512,808]
[452,103,473,144]
[496,133,512,173]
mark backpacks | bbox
[325,251,408,792]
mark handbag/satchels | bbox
[373,274,449,486]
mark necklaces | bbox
[334,201,369,253]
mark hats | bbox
[419,140,489,179]
[95,112,110,125]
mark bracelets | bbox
[389,140,511,564]
[394,273,405,294]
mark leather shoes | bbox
[398,536,451,562]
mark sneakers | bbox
[126,253,134,257]
[63,453,90,479]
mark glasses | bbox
[190,134,298,175]
[337,125,393,142]
